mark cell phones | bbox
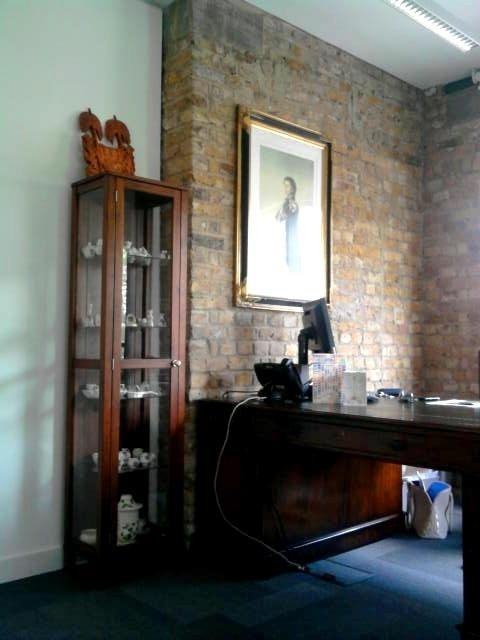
[419,396,441,401]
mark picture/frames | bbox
[231,104,332,314]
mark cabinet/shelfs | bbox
[66,171,191,591]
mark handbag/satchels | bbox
[406,471,454,540]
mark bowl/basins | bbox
[82,383,146,399]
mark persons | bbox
[275,176,303,270]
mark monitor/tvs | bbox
[302,297,335,354]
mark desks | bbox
[190,393,480,640]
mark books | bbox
[379,386,403,397]
